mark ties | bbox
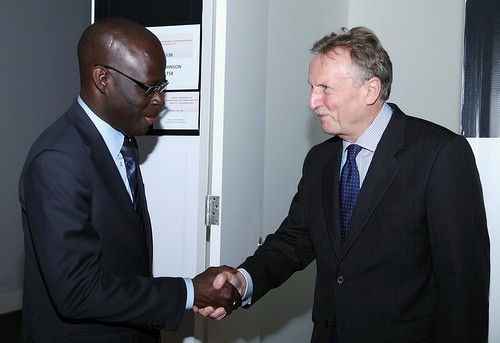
[121,137,140,210]
[338,144,363,248]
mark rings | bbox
[232,300,238,307]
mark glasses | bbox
[94,63,169,97]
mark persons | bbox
[17,14,242,343]
[192,27,490,343]
